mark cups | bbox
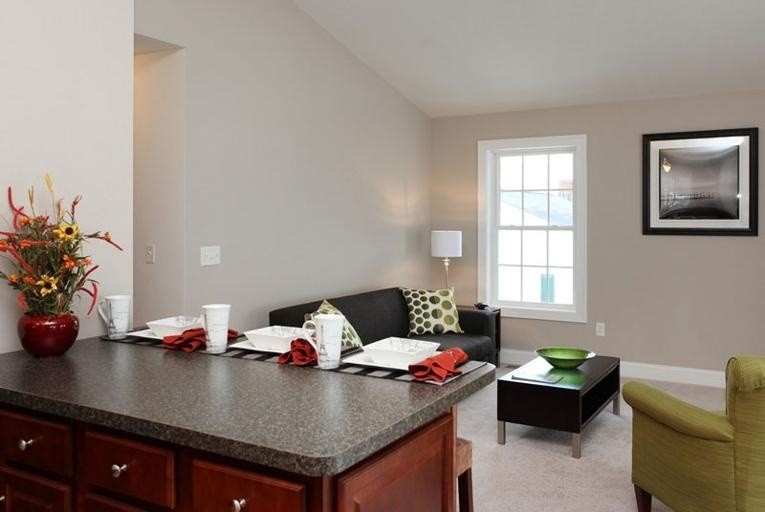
[200,304,232,353]
[302,312,345,369]
[98,295,132,340]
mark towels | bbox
[408,347,469,384]
[277,337,316,367]
[161,325,239,353]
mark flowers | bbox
[0,185,123,316]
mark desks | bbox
[455,304,501,366]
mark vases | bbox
[17,311,79,359]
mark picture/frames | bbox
[643,127,758,237]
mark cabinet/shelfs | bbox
[0,406,457,512]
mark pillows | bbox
[304,299,364,358]
[398,287,464,338]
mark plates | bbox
[341,351,441,372]
[230,340,292,356]
[124,330,165,341]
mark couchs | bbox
[268,286,500,367]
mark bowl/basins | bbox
[361,336,442,369]
[546,365,590,388]
[144,315,202,338]
[535,348,597,370]
[244,325,315,353]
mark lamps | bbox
[430,229,462,289]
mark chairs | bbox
[622,355,765,512]
